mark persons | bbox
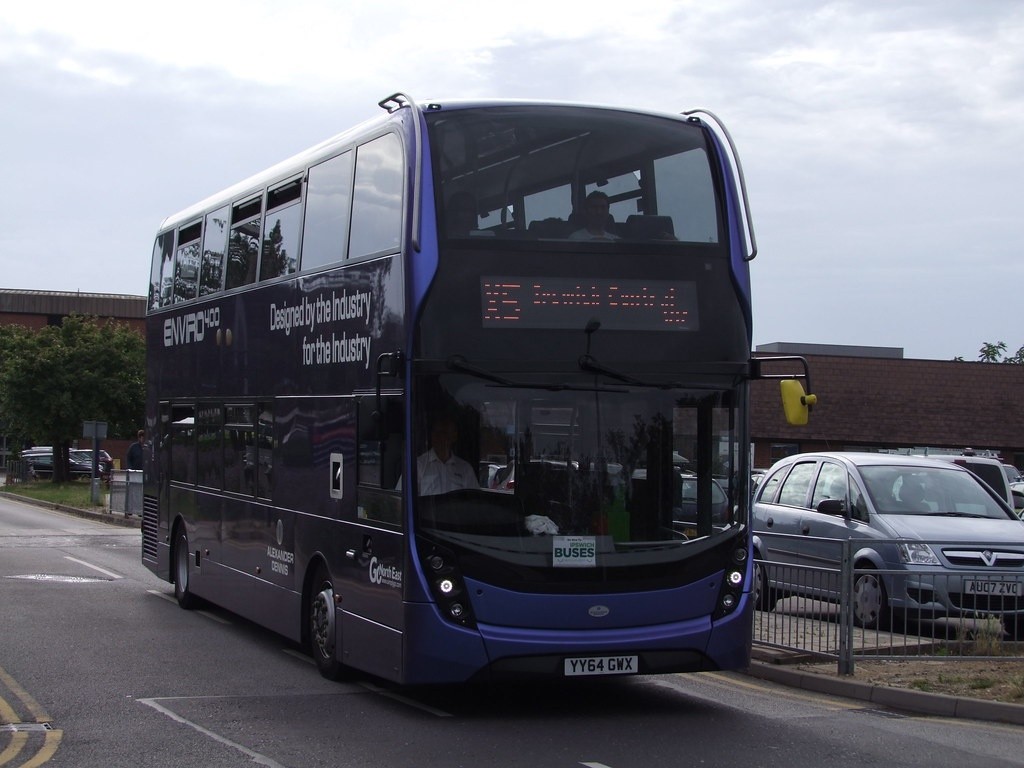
[395,414,510,495]
[127,429,145,470]
[568,192,622,239]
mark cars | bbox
[752,451,1024,642]
[675,468,768,527]
[488,458,624,492]
[892,448,1024,520]
[21,446,113,480]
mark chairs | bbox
[245,216,675,285]
[898,484,930,512]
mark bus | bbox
[141,92,820,685]
